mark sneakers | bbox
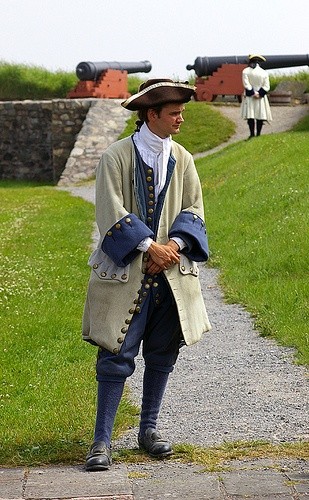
[138,427,173,456]
[85,441,112,471]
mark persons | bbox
[241,54,272,141]
[82,79,212,472]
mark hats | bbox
[248,54,266,62]
[121,79,197,111]
